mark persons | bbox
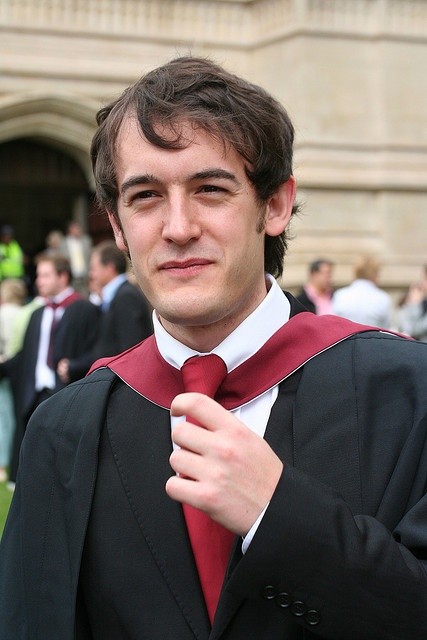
[46,288,102,370]
[1,277,43,483]
[410,263,425,343]
[296,258,333,316]
[330,254,392,331]
[0,52,426,639]
[0,226,26,282]
[55,241,153,385]
[1,252,89,491]
[42,229,65,261]
[396,280,423,338]
[63,220,95,290]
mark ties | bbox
[47,307,57,370]
[179,355,235,626]
